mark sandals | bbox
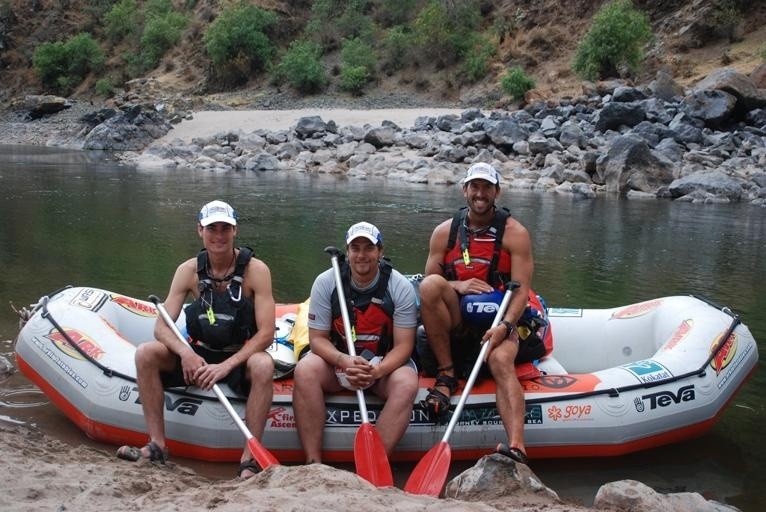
[427,372,458,414]
[116,441,166,465]
[496,442,529,464]
[237,458,259,478]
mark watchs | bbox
[497,320,514,339]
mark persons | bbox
[116,199,276,481]
[419,162,532,464]
[293,221,419,468]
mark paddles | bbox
[405,281,520,497]
[323,245,394,487]
[148,294,281,469]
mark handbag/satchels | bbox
[515,289,553,365]
[460,288,504,319]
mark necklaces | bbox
[215,253,235,291]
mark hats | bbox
[464,162,500,186]
[199,199,237,227]
[345,221,383,249]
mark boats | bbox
[15,287,759,464]
